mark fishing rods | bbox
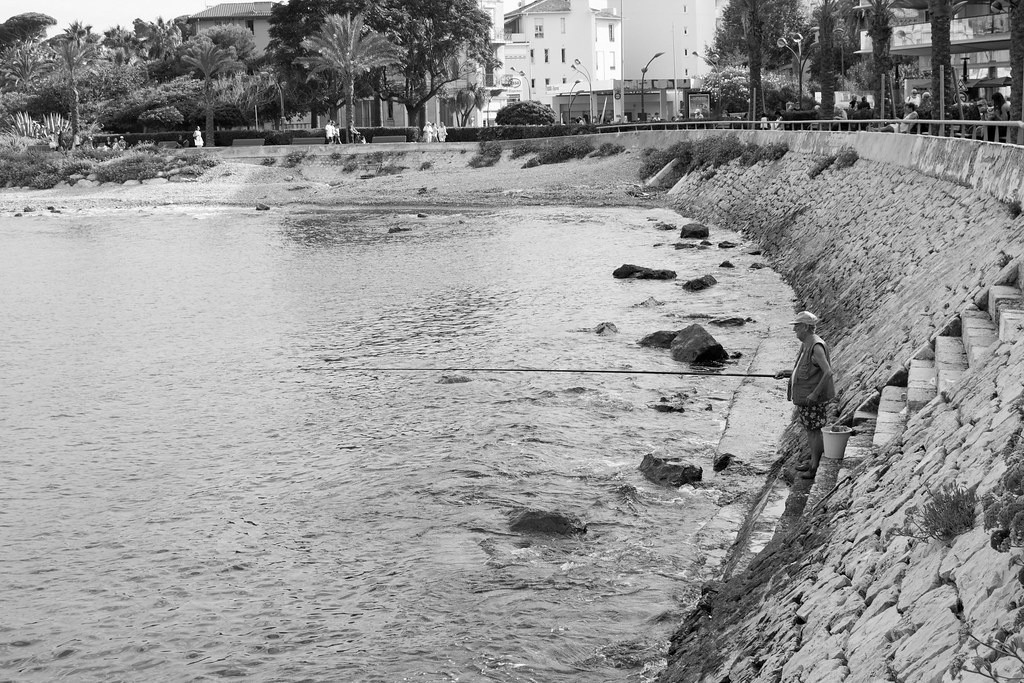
[296,368,790,378]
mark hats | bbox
[427,122,431,125]
[120,136,124,138]
[789,311,817,325]
[694,109,701,114]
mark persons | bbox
[577,109,729,124]
[119,135,126,148]
[106,136,112,147]
[775,112,785,130]
[112,137,119,149]
[325,119,358,144]
[760,112,771,130]
[178,135,187,146]
[850,88,1010,144]
[423,121,448,142]
[774,311,835,479]
[193,125,203,147]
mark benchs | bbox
[232,139,265,146]
[28,145,54,152]
[292,138,326,145]
[372,136,406,142]
[158,141,178,149]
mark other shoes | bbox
[795,462,816,480]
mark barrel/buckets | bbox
[820,416,854,461]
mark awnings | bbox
[913,78,980,88]
[978,76,1012,87]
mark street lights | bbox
[692,52,721,109]
[568,80,584,124]
[571,60,592,123]
[641,52,665,113]
[511,67,531,100]
[777,28,820,110]
